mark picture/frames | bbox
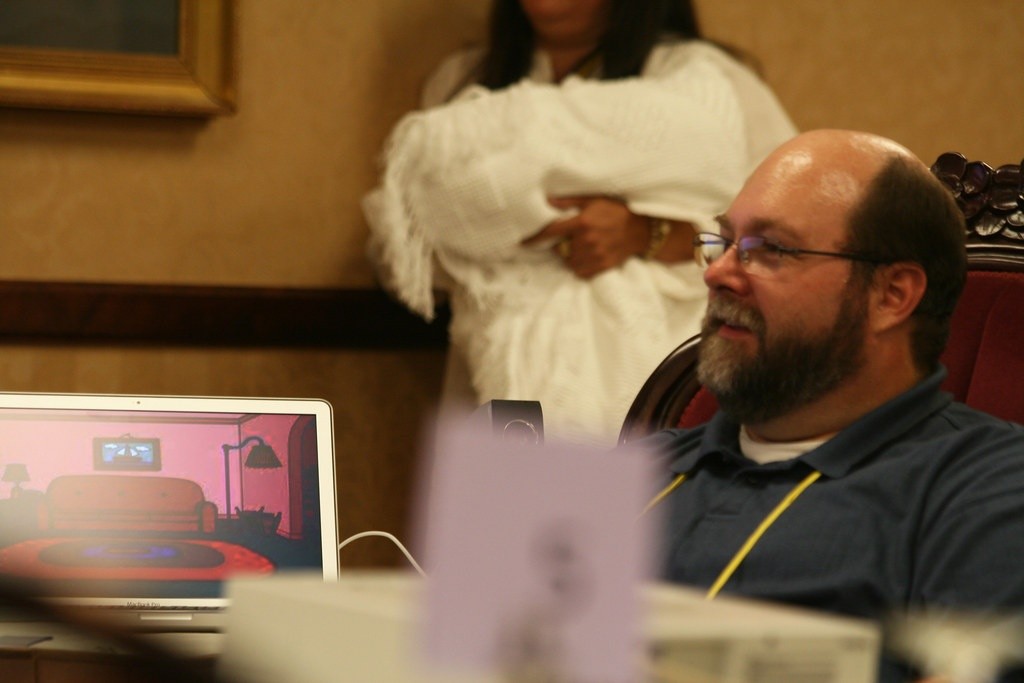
[0,0,240,116]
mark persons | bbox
[416,1,802,429]
[634,128,1021,683]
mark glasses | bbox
[693,232,884,283]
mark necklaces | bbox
[637,471,824,600]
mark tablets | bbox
[0,391,340,628]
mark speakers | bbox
[468,396,546,452]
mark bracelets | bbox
[642,216,670,262]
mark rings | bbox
[560,238,571,260]
